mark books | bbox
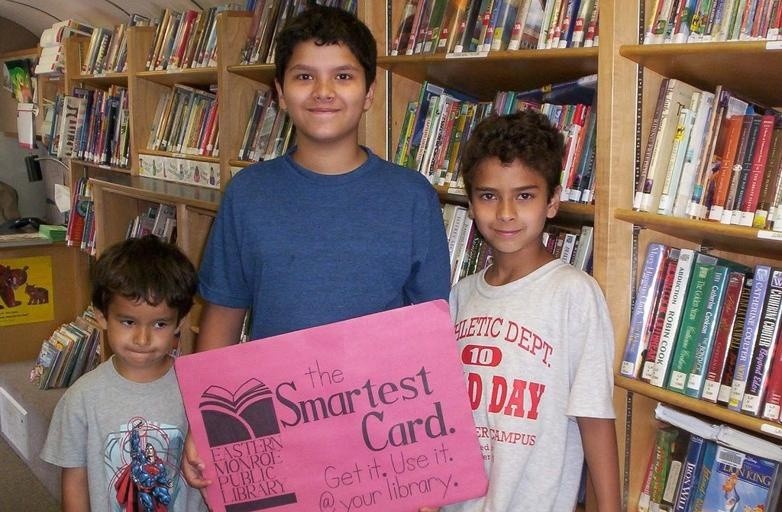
[126,202,177,244]
[32,0,357,77]
[541,226,594,272]
[632,79,781,232]
[619,242,782,425]
[393,0,599,54]
[394,74,599,206]
[32,302,100,390]
[61,176,96,257]
[48,83,220,169]
[643,1,782,45]
[237,88,297,162]
[441,203,494,288]
[636,403,781,512]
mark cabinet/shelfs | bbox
[35,1,782,511]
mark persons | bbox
[420,107,622,511]
[39,234,211,511]
[181,1,456,486]
